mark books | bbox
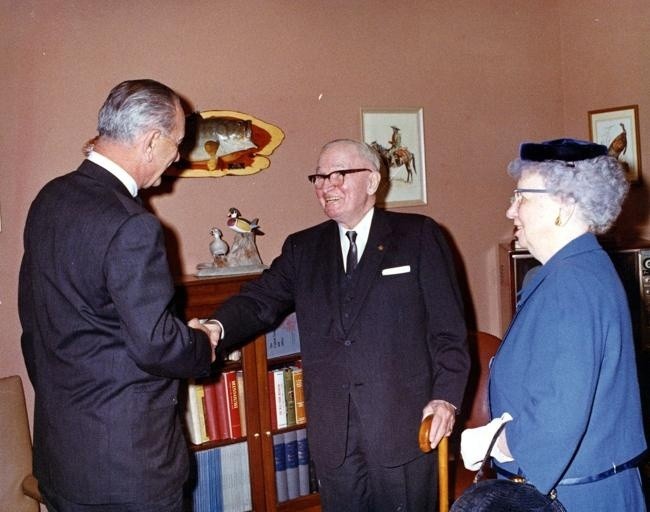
[273,429,310,503]
[182,371,247,446]
[192,440,253,512]
[268,366,307,429]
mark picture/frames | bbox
[359,106,428,209]
[587,105,643,186]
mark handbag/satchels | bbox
[448,422,566,511]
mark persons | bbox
[203,139,472,512]
[461,138,648,512]
[388,125,402,150]
[17,79,217,511]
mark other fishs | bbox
[82,110,259,171]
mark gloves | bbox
[461,413,516,471]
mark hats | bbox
[520,139,607,160]
[391,125,401,130]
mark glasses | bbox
[513,189,547,199]
[308,169,372,189]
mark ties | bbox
[346,231,357,281]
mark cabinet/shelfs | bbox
[181,276,330,512]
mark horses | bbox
[369,141,417,184]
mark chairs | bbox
[0,376,44,512]
